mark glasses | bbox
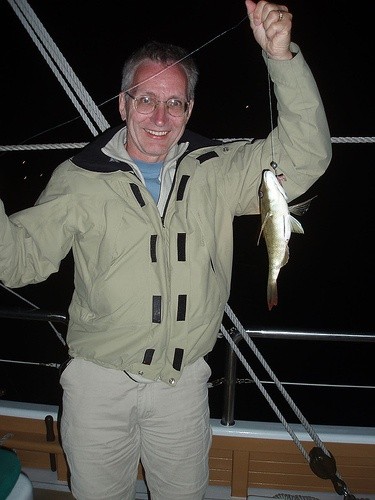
[126,92,190,117]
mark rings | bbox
[277,10,283,22]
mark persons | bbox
[0,0,332,500]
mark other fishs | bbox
[255,167,308,312]
